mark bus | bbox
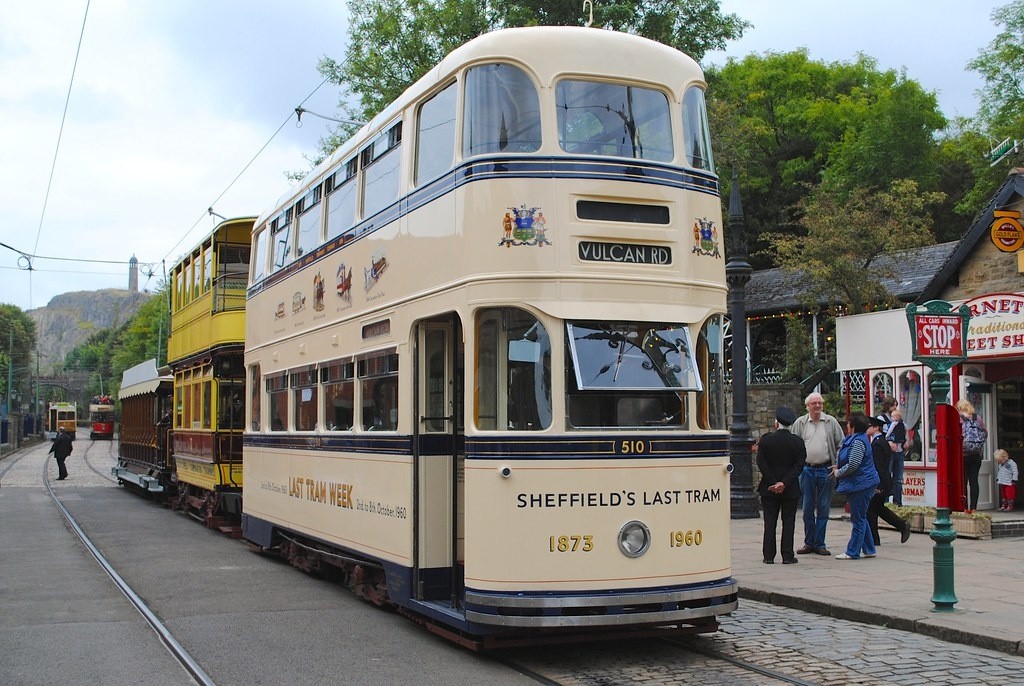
[48,402,78,441]
[240,26,741,656]
[88,403,115,440]
[109,216,262,535]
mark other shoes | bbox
[1003,505,1014,512]
[901,522,910,543]
[64,473,68,478]
[782,557,798,564]
[763,557,774,564]
[997,504,1007,511]
[797,545,815,554]
[859,552,876,558]
[834,553,858,559]
[814,548,831,555]
[55,475,64,480]
[873,538,880,545]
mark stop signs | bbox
[914,312,967,358]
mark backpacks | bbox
[960,413,985,454]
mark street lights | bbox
[31,396,43,437]
[10,390,23,448]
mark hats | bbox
[867,416,886,427]
[775,407,797,426]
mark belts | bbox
[804,461,831,469]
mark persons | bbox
[790,392,845,556]
[865,416,910,546]
[885,409,909,453]
[833,411,880,559]
[93,394,114,405]
[877,397,908,507]
[156,394,173,446]
[49,426,73,480]
[756,406,807,565]
[993,449,1018,512]
[955,399,985,513]
